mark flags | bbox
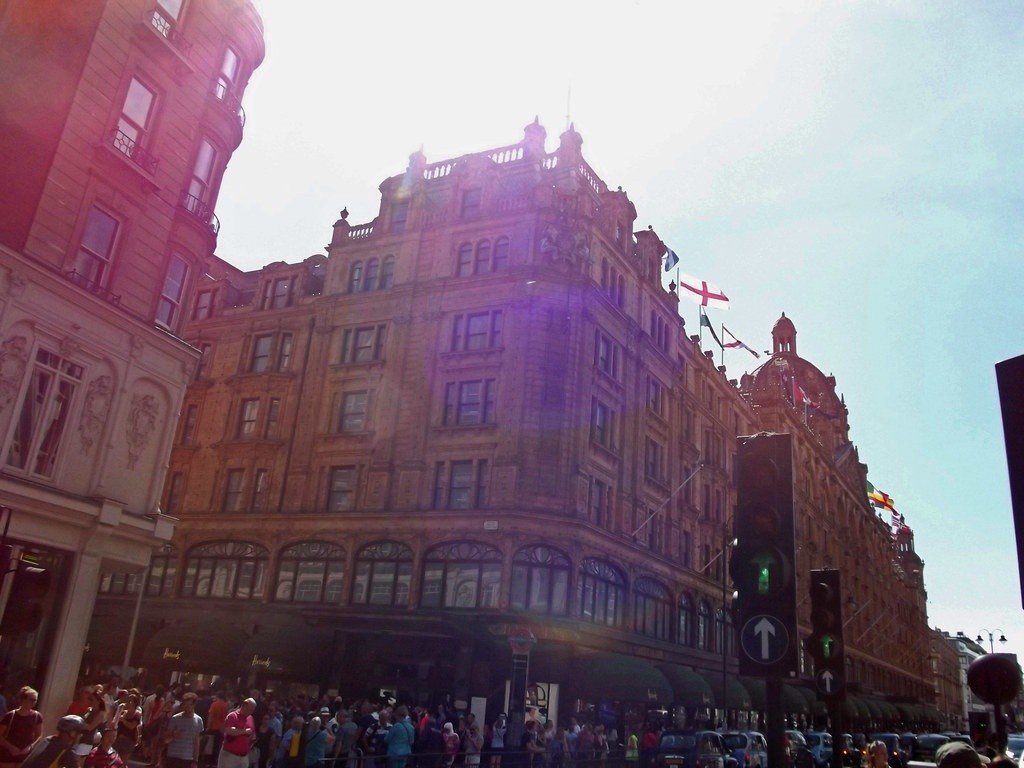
[700,306,724,351]
[666,247,679,273]
[679,272,731,312]
[723,323,761,359]
[867,481,912,534]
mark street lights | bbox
[976,627,1007,652]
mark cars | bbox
[653,725,1024,768]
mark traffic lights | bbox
[728,434,795,610]
[807,569,844,669]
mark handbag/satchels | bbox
[368,733,385,755]
[199,735,214,755]
[599,738,607,752]
[625,750,638,760]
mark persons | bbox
[0,668,1024,768]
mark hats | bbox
[935,741,991,767]
[57,715,88,731]
[321,707,329,715]
